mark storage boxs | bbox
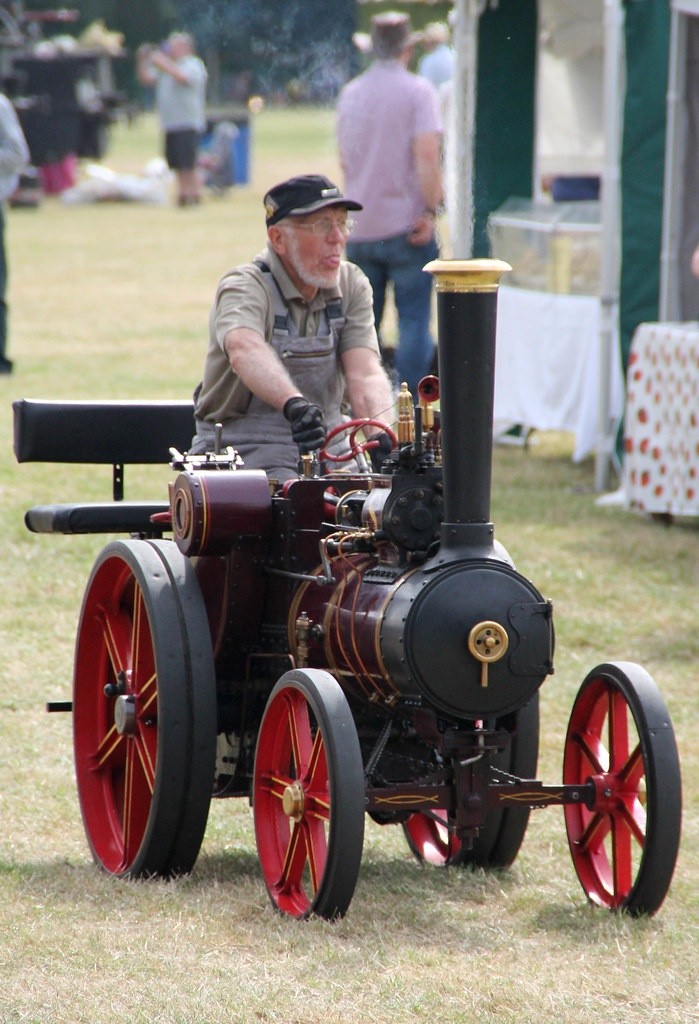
[488,194,602,295]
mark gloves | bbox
[366,430,396,472]
[281,393,328,459]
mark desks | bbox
[596,315,698,523]
[484,285,601,461]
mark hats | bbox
[370,8,414,41]
[261,174,364,225]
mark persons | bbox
[189,174,395,489]
[0,94,30,372]
[422,25,456,93]
[138,32,206,204]
[336,13,444,403]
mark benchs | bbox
[12,395,198,534]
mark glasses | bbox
[282,215,359,236]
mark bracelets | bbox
[423,208,436,216]
